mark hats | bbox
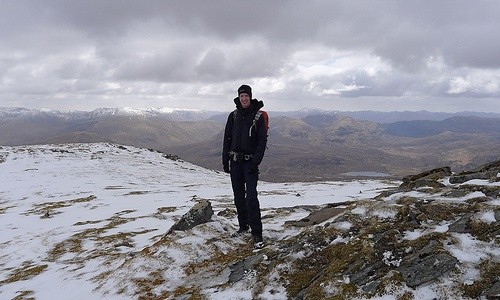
[238,85,252,98]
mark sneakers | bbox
[229,225,249,238]
[253,234,264,249]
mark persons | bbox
[222,85,267,242]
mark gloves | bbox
[222,162,231,173]
